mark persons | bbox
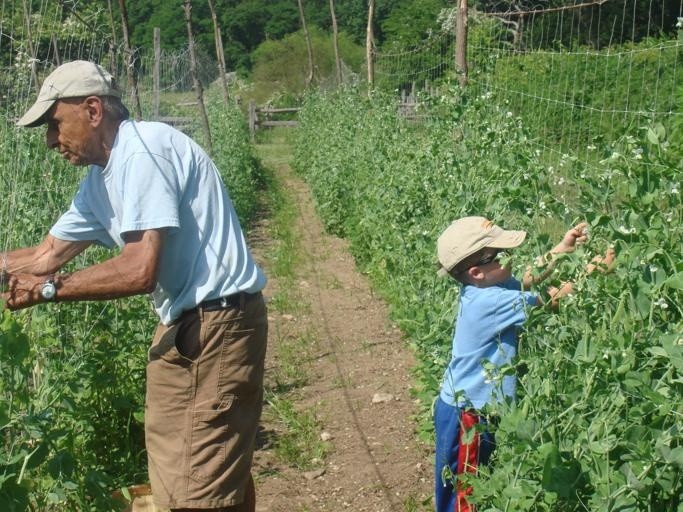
[1,57,268,512]
[434,215,615,512]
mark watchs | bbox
[42,273,55,303]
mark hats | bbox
[435,215,527,278]
[14,58,123,128]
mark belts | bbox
[183,290,261,315]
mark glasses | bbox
[453,248,514,276]
